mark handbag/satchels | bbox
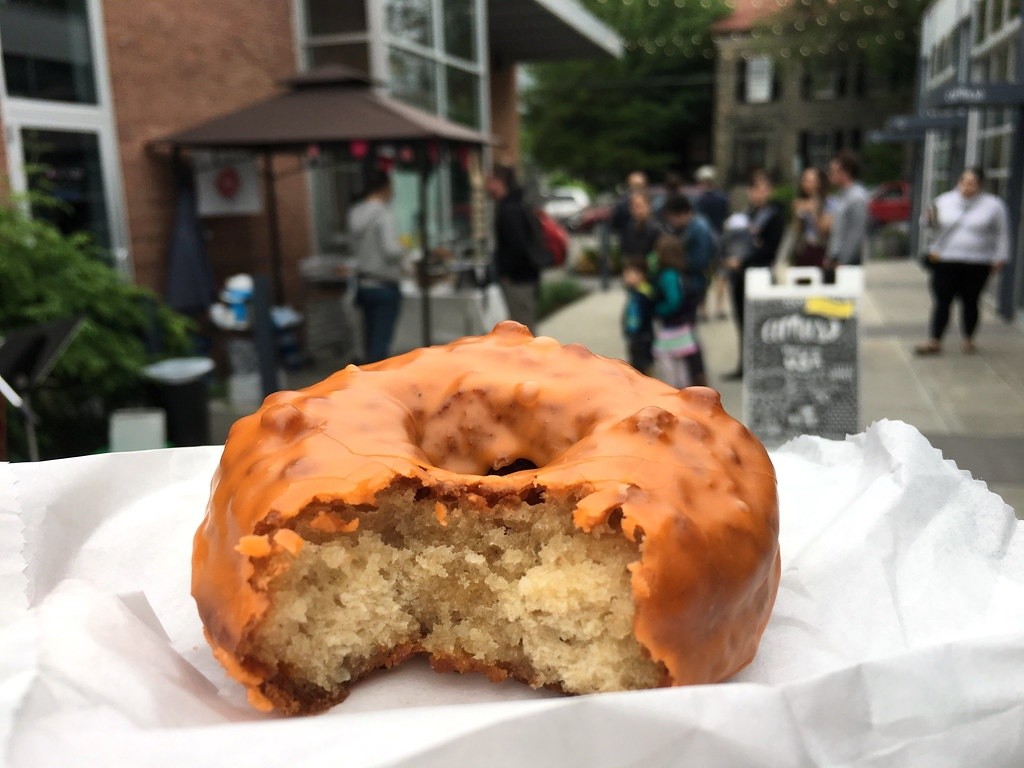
[651,317,699,359]
[921,253,939,270]
[622,288,642,332]
[527,203,567,264]
[696,278,740,376]
[482,262,509,331]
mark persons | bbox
[914,167,1012,354]
[348,149,872,388]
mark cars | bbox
[544,185,616,262]
[861,180,913,234]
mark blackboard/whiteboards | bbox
[745,264,861,448]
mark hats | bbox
[695,165,716,180]
[724,212,749,230]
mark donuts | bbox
[188,317,782,714]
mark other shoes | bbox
[961,339,974,350]
[913,344,940,355]
